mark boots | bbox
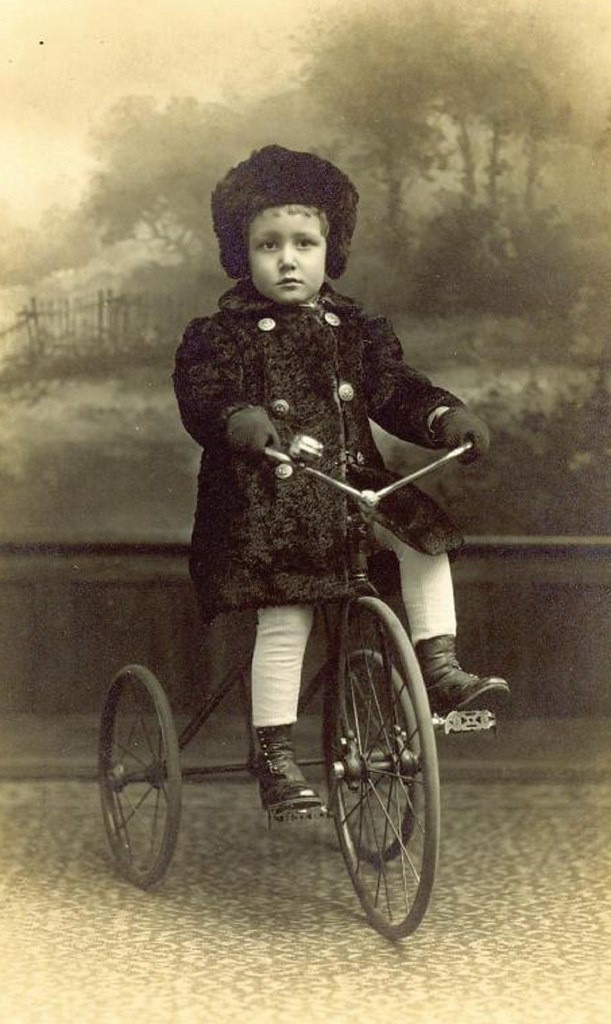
[254,722,323,813]
[414,634,513,715]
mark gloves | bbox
[224,405,281,451]
[435,406,490,466]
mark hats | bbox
[210,145,360,280]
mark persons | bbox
[171,144,510,825]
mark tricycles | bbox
[96,428,502,940]
